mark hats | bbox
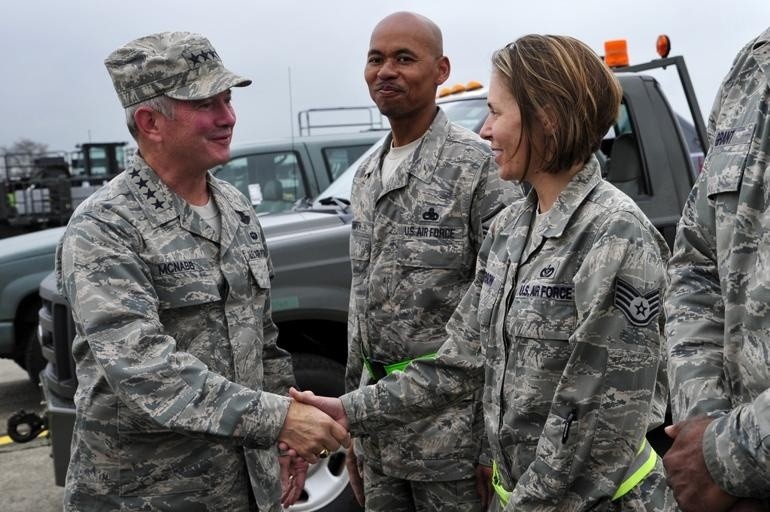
[105,32,252,109]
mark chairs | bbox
[252,179,286,217]
[606,134,642,196]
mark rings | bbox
[317,448,329,458]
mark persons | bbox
[344,12,525,512]
[54,30,351,512]
[279,34,679,512]
[662,27,770,512]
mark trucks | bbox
[39,34,711,512]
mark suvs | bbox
[1,129,391,391]
